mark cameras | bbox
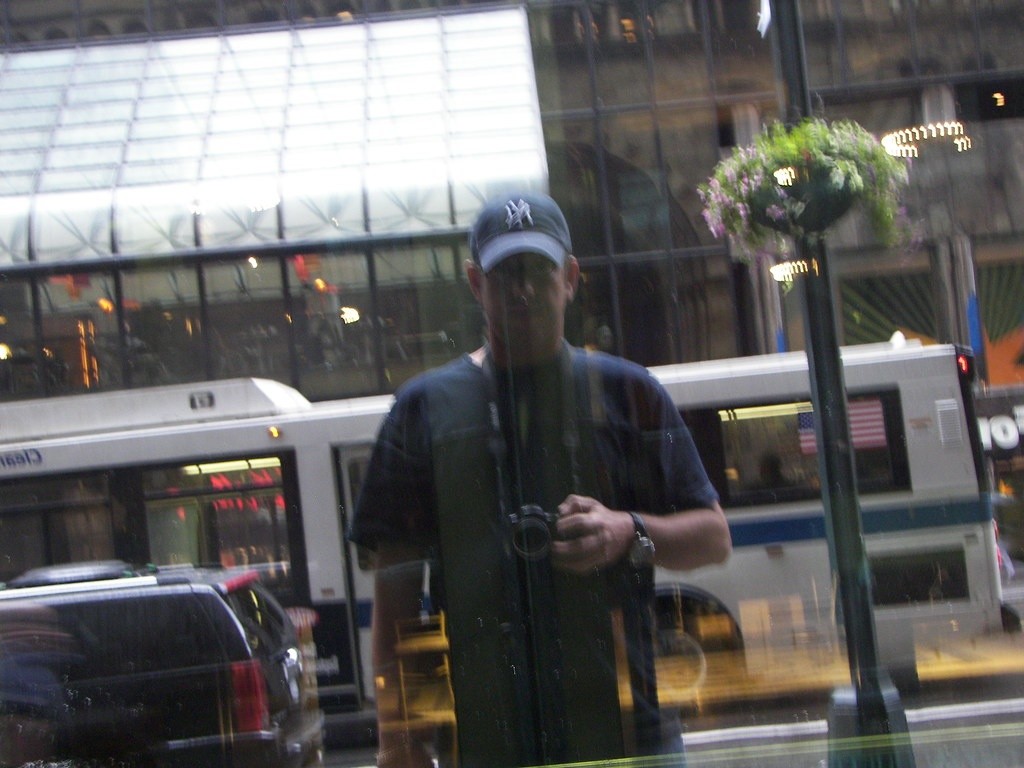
[510,503,566,562]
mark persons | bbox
[345,189,731,768]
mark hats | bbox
[468,185,572,275]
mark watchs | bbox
[627,511,655,576]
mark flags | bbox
[799,401,887,455]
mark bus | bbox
[1,339,1004,737]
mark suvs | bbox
[1,558,324,766]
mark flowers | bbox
[696,91,926,296]
